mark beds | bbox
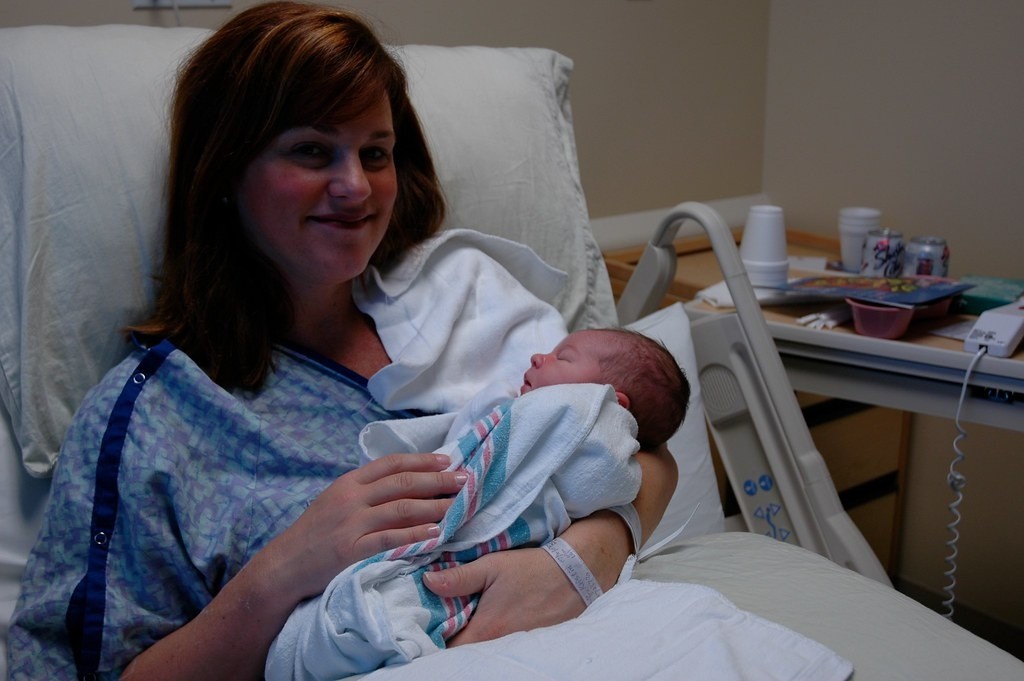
[0,200,1024,681]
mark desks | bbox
[684,250,1024,434]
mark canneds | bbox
[902,236,950,276]
[857,227,906,278]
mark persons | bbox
[0,0,678,681]
[266,324,689,680]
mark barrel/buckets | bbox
[838,206,880,272]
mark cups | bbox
[741,206,787,297]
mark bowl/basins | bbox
[914,298,952,319]
[845,298,915,338]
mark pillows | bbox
[0,26,623,476]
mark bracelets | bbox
[609,503,699,561]
[543,536,636,612]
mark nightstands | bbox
[604,221,912,591]
